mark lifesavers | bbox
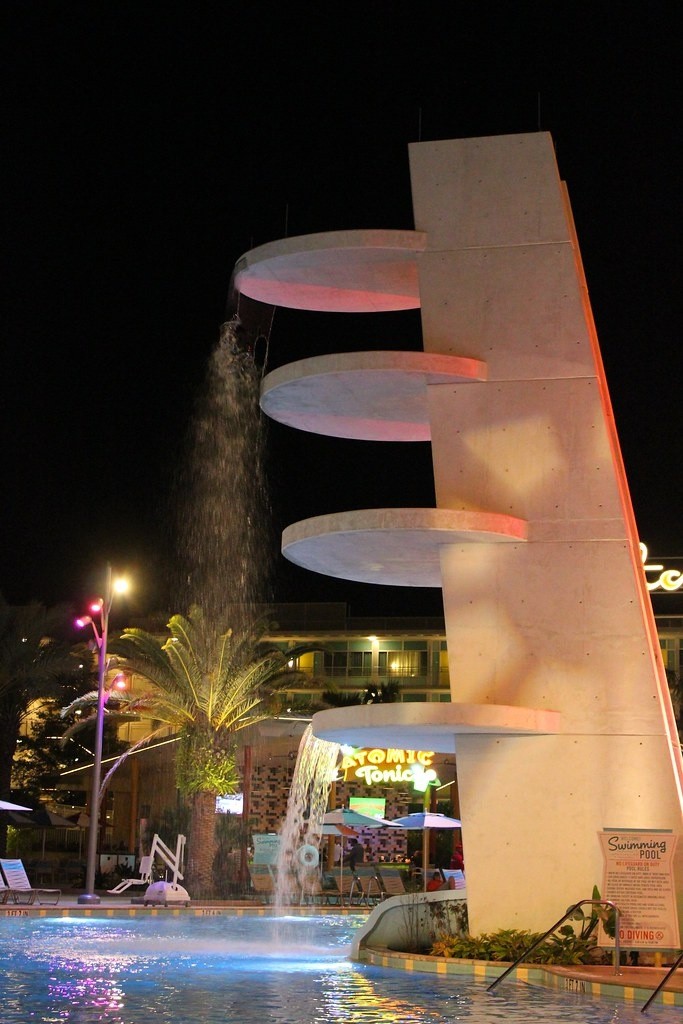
[296,845,320,869]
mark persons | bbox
[362,843,393,863]
[427,871,455,892]
[448,844,464,870]
[319,848,327,862]
[342,840,365,872]
[332,840,343,867]
[412,850,422,869]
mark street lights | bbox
[74,561,130,905]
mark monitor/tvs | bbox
[349,797,386,819]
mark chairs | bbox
[0,858,61,905]
[419,869,467,891]
[107,855,154,894]
[246,865,407,907]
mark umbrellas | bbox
[386,810,462,892]
[305,824,361,877]
[0,800,34,812]
[303,807,404,903]
